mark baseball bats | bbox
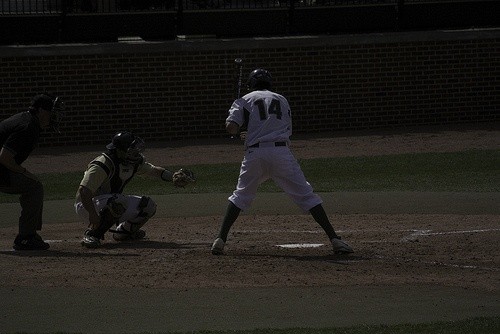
[230,58,242,139]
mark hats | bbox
[29,93,53,111]
[106,132,134,151]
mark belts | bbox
[251,140,287,148]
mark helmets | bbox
[247,69,274,91]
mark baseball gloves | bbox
[172,168,195,186]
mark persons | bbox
[74,131,196,249]
[0,94,65,250]
[211,68,355,256]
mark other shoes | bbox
[211,238,225,254]
[81,229,97,247]
[13,234,49,250]
[113,226,145,241]
[331,239,353,253]
